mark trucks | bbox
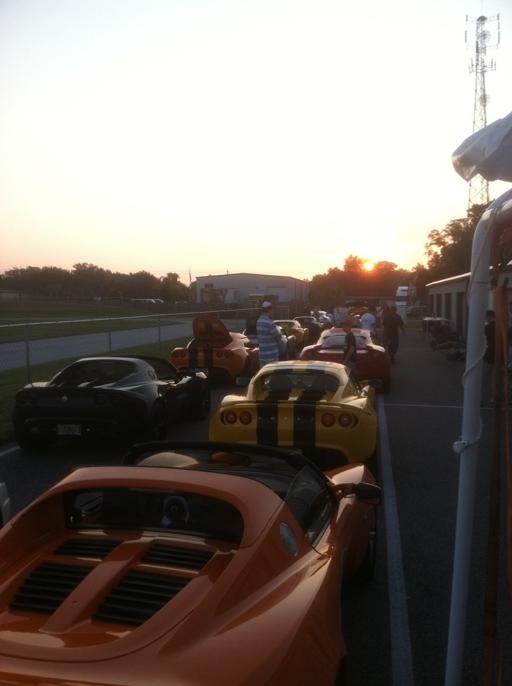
[393,286,417,310]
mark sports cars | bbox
[11,355,207,453]
[169,312,261,387]
[275,297,391,349]
[207,360,379,472]
[0,439,384,686]
[297,326,391,390]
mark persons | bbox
[342,303,408,394]
[256,301,284,384]
[484,309,496,406]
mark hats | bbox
[262,301,274,308]
[340,318,352,326]
